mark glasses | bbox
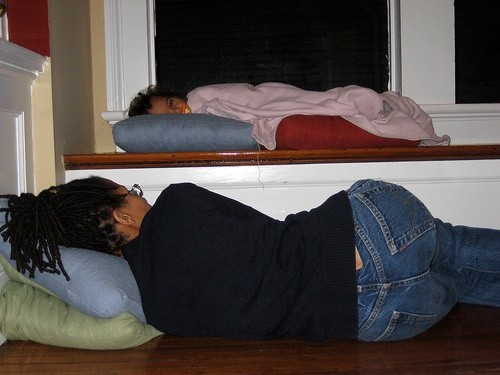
[122,184,143,200]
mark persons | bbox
[130,85,193,118]
[0,178,499,341]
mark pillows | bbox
[276,115,421,149]
[112,113,261,151]
[0,199,164,350]
